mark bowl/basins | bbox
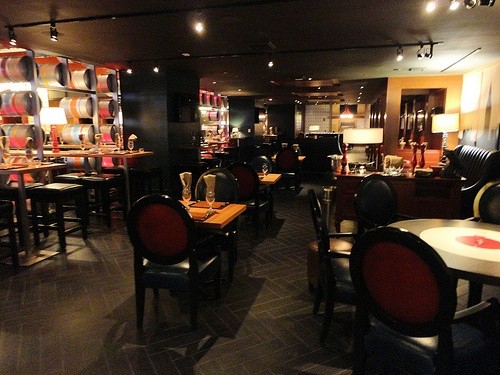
[415,166,444,177]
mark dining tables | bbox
[271,155,306,190]
[253,172,283,240]
[0,162,66,268]
[9,149,153,236]
[178,202,248,300]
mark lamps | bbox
[7,0,495,74]
[432,113,460,161]
[340,107,354,118]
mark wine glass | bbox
[262,165,268,177]
[182,188,191,209]
[0,138,135,166]
[206,191,215,212]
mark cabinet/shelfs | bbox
[370,92,427,140]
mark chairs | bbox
[0,146,500,375]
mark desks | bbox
[387,218,500,375]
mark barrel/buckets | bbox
[0,55,119,182]
[199,93,227,135]
